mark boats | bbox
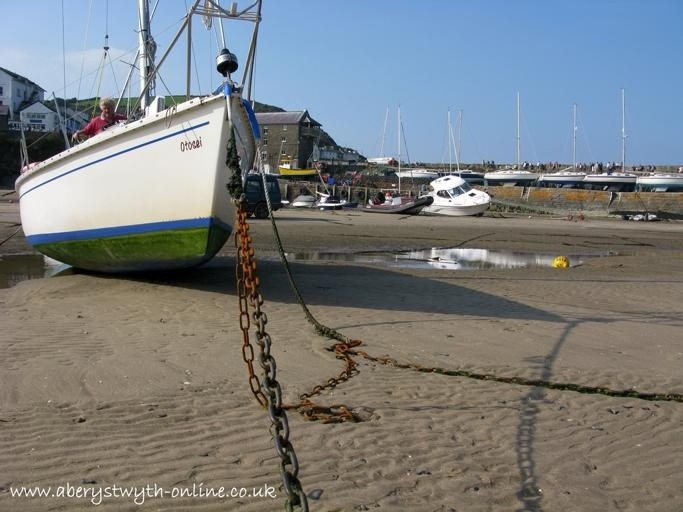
[279,158,322,176]
[637,172,683,191]
[293,190,359,209]
[423,174,491,217]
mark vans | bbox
[237,173,281,218]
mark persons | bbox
[328,175,359,186]
[73,96,127,140]
[393,190,399,197]
[377,192,385,204]
[482,159,656,173]
[20,147,44,173]
[385,189,391,196]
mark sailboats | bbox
[540,104,584,187]
[15,1,262,275]
[361,104,434,213]
[367,106,398,166]
[486,89,539,186]
[585,90,636,191]
[444,107,484,184]
[395,108,439,178]
